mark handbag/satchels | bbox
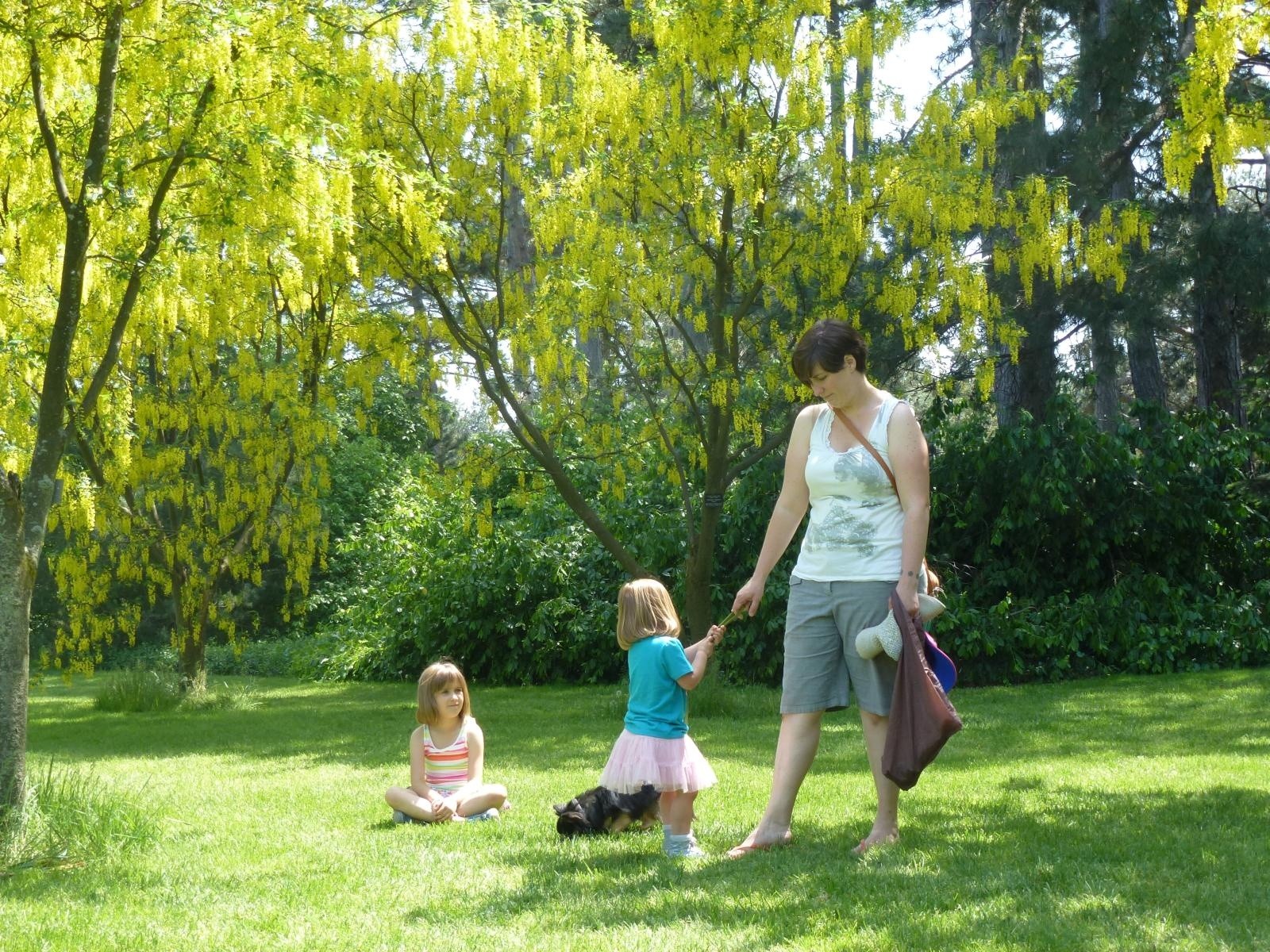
[923,559,940,599]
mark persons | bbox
[385,661,507,825]
[726,321,930,861]
[599,579,727,860]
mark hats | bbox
[924,631,957,694]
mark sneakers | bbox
[393,811,413,824]
[464,807,501,823]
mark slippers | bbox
[726,844,793,861]
[854,834,900,853]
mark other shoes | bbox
[662,825,671,855]
[667,829,707,857]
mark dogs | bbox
[553,784,660,836]
